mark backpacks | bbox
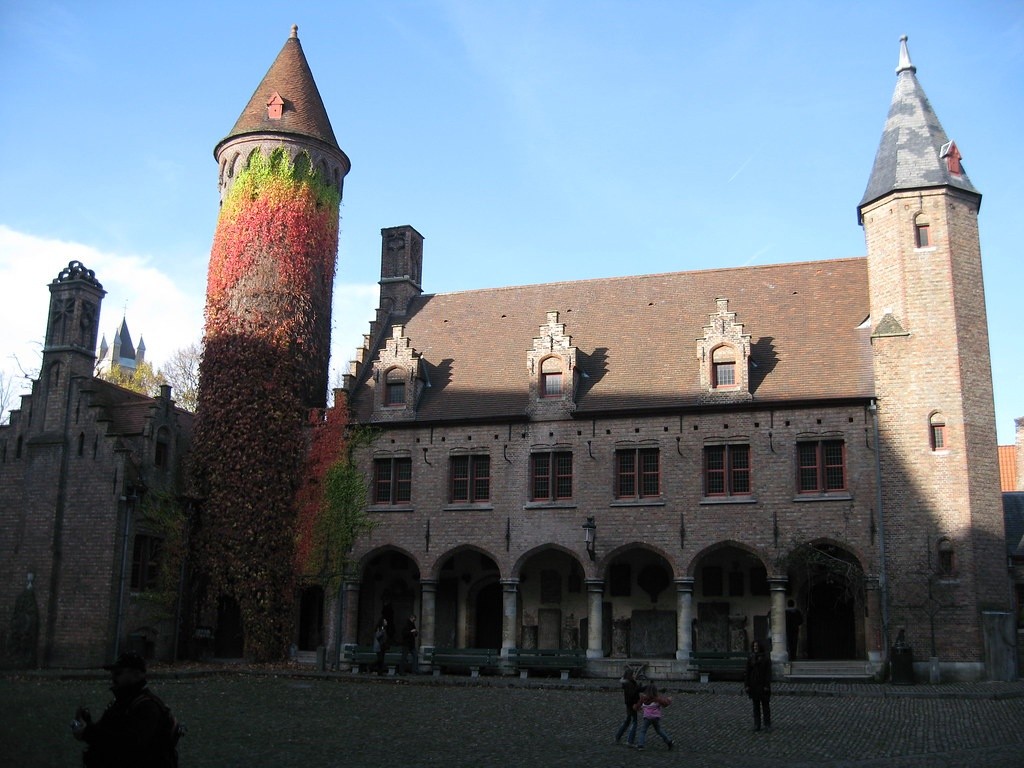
[126,692,188,768]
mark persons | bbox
[399,614,423,676]
[785,599,803,659]
[745,639,773,732]
[378,594,396,644]
[370,617,389,676]
[72,650,180,768]
[633,685,675,750]
[614,670,654,747]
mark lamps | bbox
[582,515,596,562]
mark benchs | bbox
[345,645,413,675]
[423,647,499,678]
[503,648,587,679]
[687,652,752,684]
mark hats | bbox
[103,651,146,673]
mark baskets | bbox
[636,674,648,693]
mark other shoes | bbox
[636,745,645,750]
[752,725,760,731]
[668,740,675,751]
[626,742,638,748]
[614,737,624,745]
